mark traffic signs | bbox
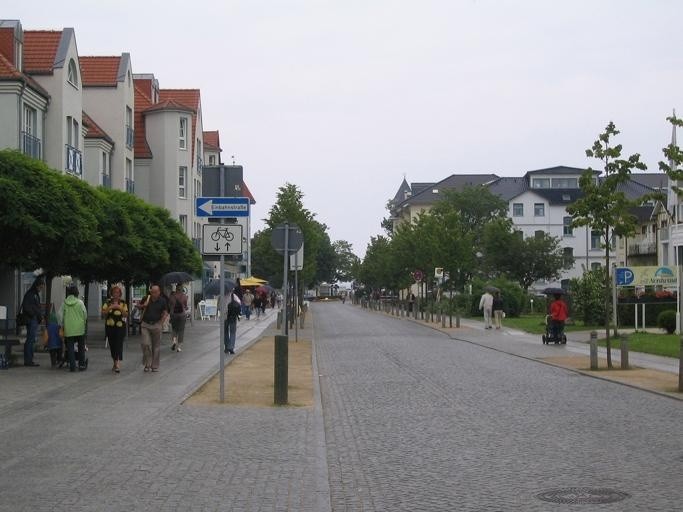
[197,197,249,218]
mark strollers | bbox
[131,305,140,335]
[54,327,89,370]
[542,315,566,345]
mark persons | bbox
[244,289,283,318]
[216,291,243,354]
[100,284,129,373]
[479,290,493,328]
[550,293,568,345]
[492,291,504,329]
[57,284,88,371]
[243,290,284,319]
[167,283,189,353]
[21,278,45,366]
[136,284,168,372]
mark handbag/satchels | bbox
[227,300,241,323]
[16,313,29,326]
[131,306,145,324]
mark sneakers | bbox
[144,367,159,372]
[112,365,120,372]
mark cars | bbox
[303,294,315,301]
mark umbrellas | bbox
[201,277,240,294]
[158,270,193,285]
[240,276,275,289]
[542,285,568,295]
[481,284,501,293]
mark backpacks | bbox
[173,294,184,313]
